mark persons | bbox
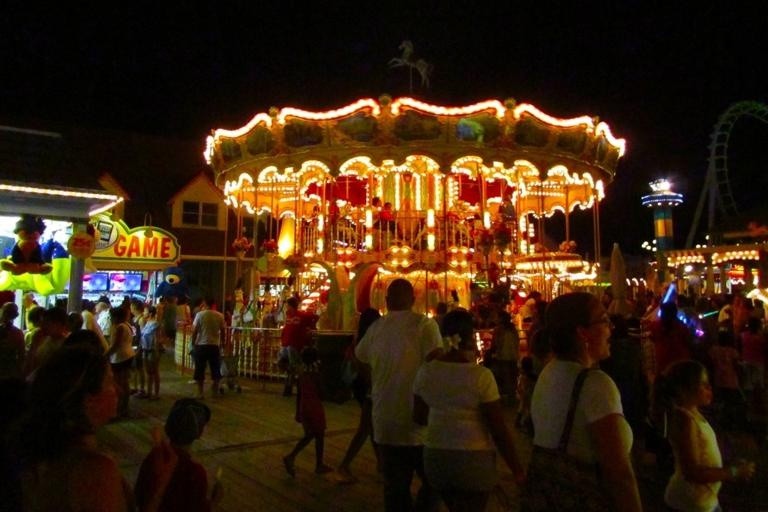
[498,194,516,220]
[0,278,768,512]
[329,204,365,243]
[372,197,410,241]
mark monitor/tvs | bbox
[109,273,143,292]
[82,271,109,293]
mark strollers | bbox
[214,342,242,397]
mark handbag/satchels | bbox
[518,443,610,511]
